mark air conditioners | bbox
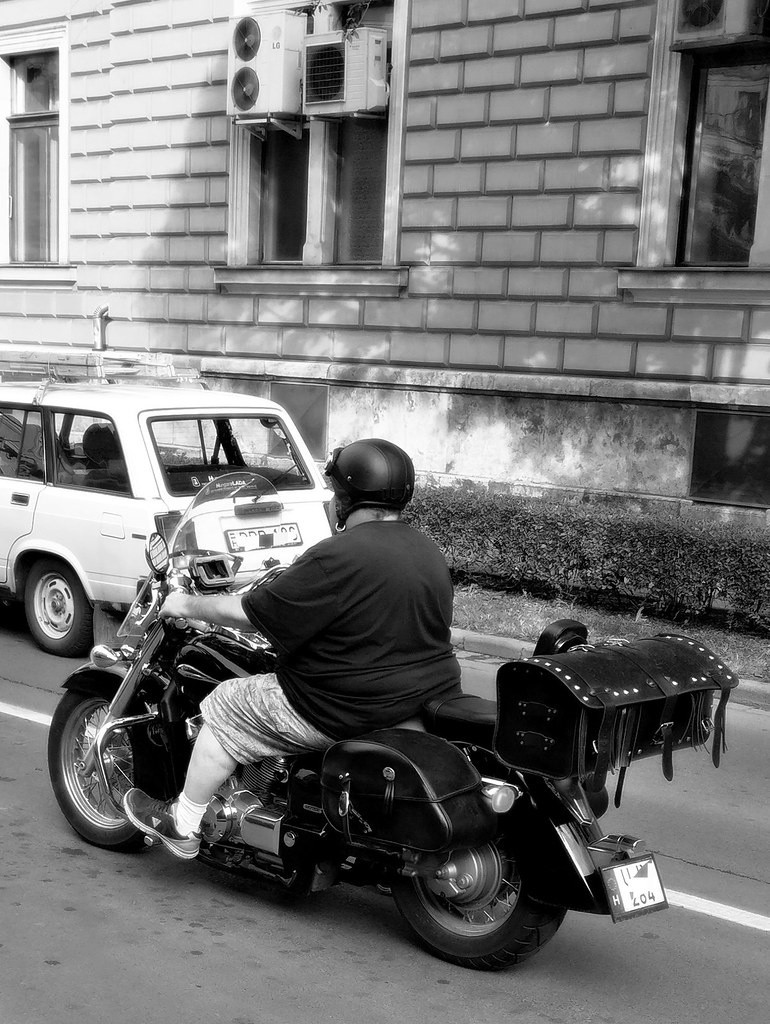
[301,28,388,119]
[673,0,763,46]
[226,9,308,120]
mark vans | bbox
[0,381,334,662]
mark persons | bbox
[124,439,463,859]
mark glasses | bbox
[323,448,342,476]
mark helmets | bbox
[330,438,415,519]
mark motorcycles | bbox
[47,471,739,972]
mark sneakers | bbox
[123,788,203,859]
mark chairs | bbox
[82,423,130,493]
